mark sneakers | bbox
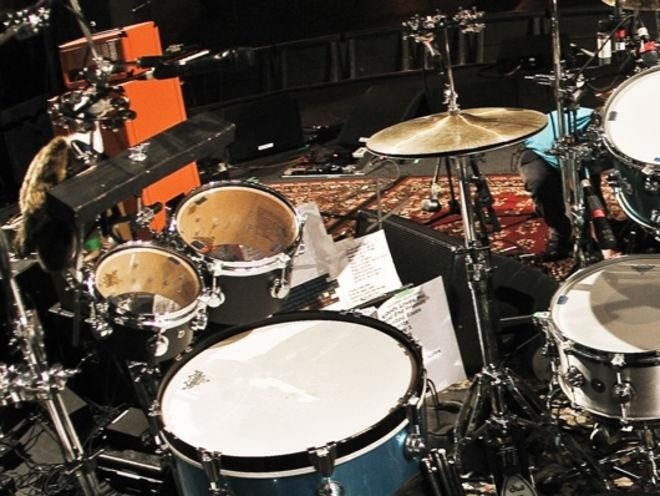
[573,238,600,263]
[545,239,568,260]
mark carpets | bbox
[246,168,660,288]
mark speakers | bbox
[220,90,305,166]
[355,208,556,380]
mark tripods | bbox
[420,60,483,241]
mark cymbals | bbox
[366,108,550,157]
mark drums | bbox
[151,310,429,495]
[546,255,660,422]
[85,240,205,365]
[594,66,660,230]
[169,180,305,328]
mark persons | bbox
[518,105,602,262]
